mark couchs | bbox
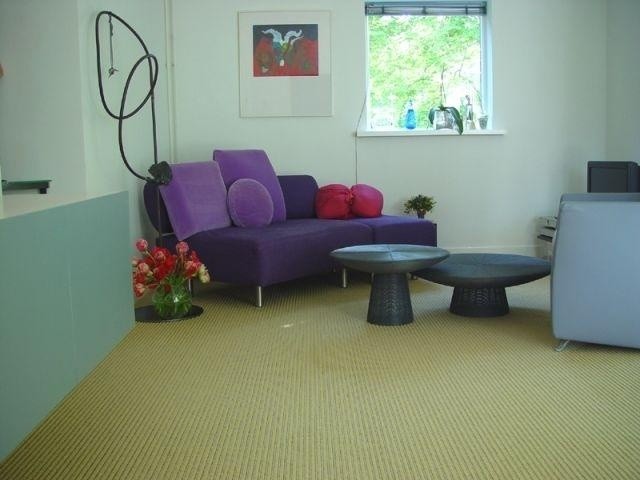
[142,174,437,309]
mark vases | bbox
[150,278,194,319]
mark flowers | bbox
[403,192,437,220]
[129,237,214,317]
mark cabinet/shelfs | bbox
[537,214,558,259]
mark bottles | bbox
[405,100,416,129]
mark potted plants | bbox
[465,110,478,131]
[429,103,465,135]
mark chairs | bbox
[546,190,640,353]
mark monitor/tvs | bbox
[587,161,640,192]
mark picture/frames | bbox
[237,9,334,117]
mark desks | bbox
[327,242,452,328]
[406,252,553,318]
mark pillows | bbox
[212,146,288,225]
[156,159,233,242]
[315,182,386,222]
[224,178,275,228]
